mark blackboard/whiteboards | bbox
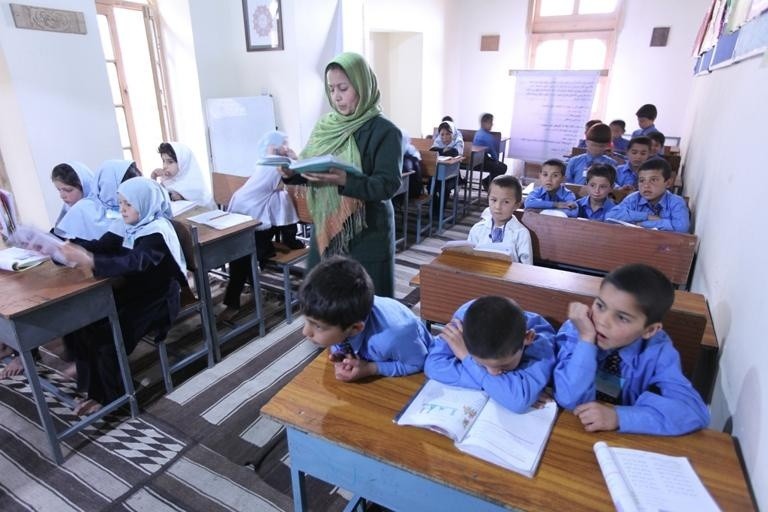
[205,95,277,176]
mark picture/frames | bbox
[242,0,283,54]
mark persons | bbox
[299,255,435,382]
[431,113,508,192]
[525,104,691,232]
[265,52,401,299]
[467,176,533,265]
[552,263,710,436]
[0,129,307,417]
[420,292,556,414]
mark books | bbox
[591,440,722,512]
[256,153,365,183]
[392,375,564,479]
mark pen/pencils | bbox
[209,213,230,221]
